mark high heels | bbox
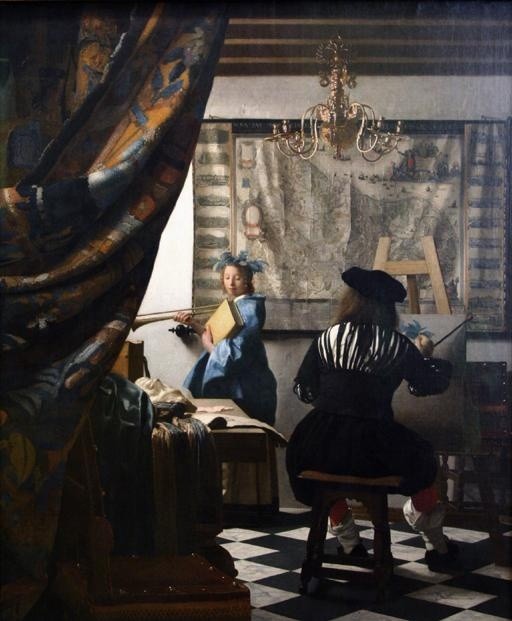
[334,543,369,567]
[425,542,459,570]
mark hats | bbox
[342,267,407,303]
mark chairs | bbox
[51,412,252,620]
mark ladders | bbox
[371,235,502,548]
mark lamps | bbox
[265,26,409,162]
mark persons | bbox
[172,249,278,425]
[284,266,462,573]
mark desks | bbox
[177,397,269,578]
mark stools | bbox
[207,392,279,513]
[296,467,405,603]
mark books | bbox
[203,299,244,344]
